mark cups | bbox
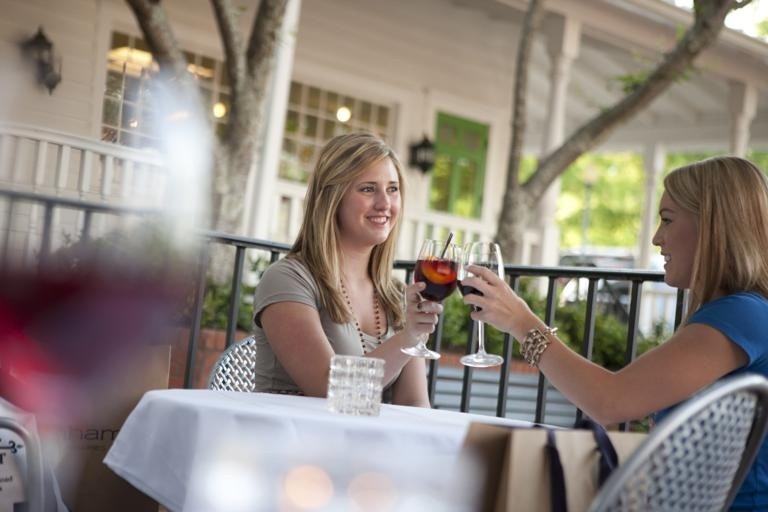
[326,356,385,418]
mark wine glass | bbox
[0,52,208,506]
[399,240,458,360]
[457,241,506,369]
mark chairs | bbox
[578,371,767,512]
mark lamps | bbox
[408,133,437,175]
[21,25,63,96]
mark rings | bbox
[417,303,422,309]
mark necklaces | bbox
[337,274,381,355]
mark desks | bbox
[140,388,578,511]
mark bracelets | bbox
[518,324,558,369]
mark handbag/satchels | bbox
[54,334,171,512]
[456,417,650,512]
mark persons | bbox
[251,130,444,412]
[461,156,768,511]
